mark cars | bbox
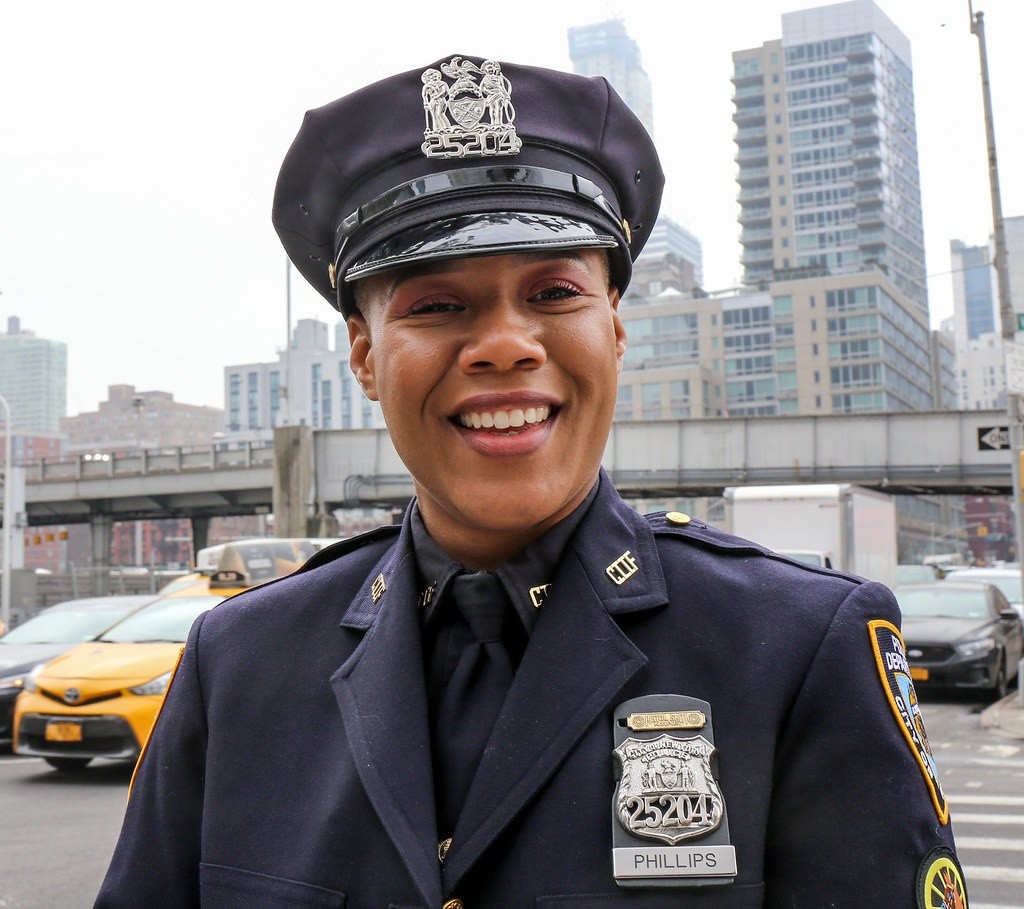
[156,573,208,598]
[0,596,158,756]
[946,568,1023,620]
[893,581,1024,702]
[894,564,946,586]
[13,587,251,771]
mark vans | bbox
[926,555,964,578]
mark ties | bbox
[431,570,516,842]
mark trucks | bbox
[723,484,899,582]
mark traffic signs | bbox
[978,427,1010,452]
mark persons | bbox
[91,52,971,909]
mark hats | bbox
[271,55,665,321]
